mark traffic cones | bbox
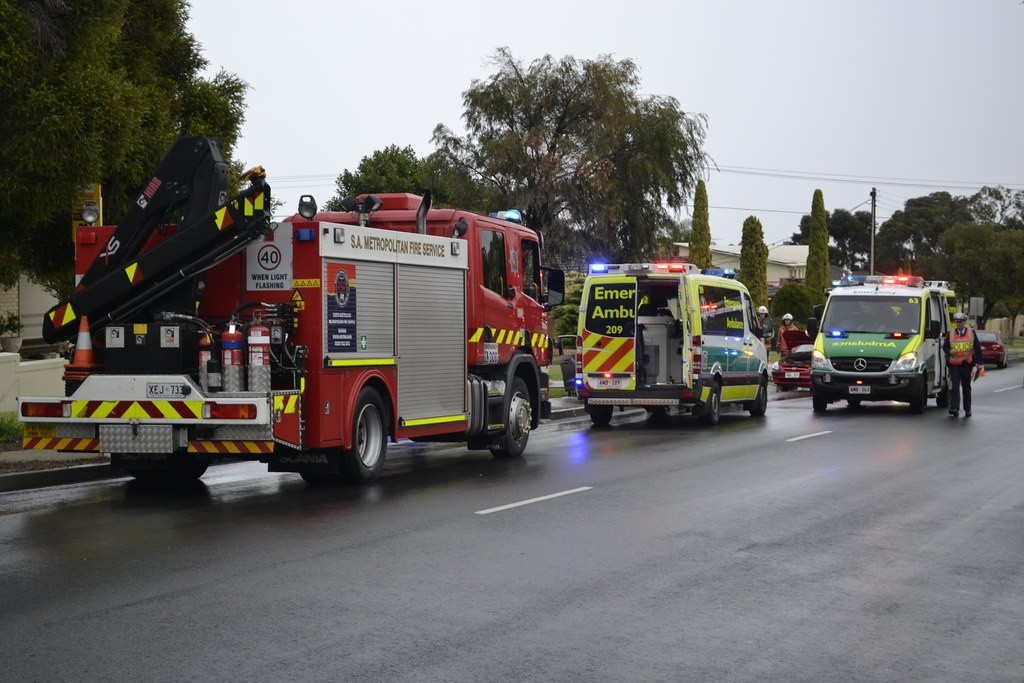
[63,316,104,380]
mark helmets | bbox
[782,313,793,320]
[758,306,768,313]
[954,313,968,322]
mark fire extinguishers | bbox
[244,317,270,365]
[221,313,244,366]
[186,320,221,368]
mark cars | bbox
[975,330,1008,368]
[771,329,816,392]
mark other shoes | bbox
[966,411,972,416]
[949,408,959,417]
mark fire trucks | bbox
[16,132,565,485]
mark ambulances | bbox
[558,263,772,424]
[807,275,959,413]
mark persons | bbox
[942,313,982,417]
[758,306,771,360]
[776,313,799,357]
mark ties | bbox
[960,332,962,337]
